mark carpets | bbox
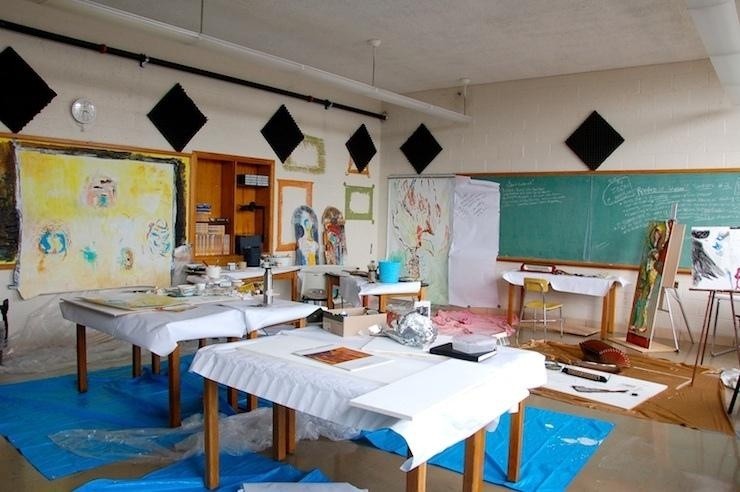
[0,346,360,478]
[348,398,614,492]
[68,445,356,490]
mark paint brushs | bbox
[368,265,379,271]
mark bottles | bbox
[227,263,235,271]
[263,268,274,305]
[370,261,376,271]
[232,280,242,288]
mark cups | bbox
[236,261,247,271]
[368,269,378,283]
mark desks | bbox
[503,269,618,341]
[60,283,309,429]
[187,327,545,491]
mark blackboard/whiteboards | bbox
[456,167,740,274]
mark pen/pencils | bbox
[202,260,208,267]
[215,259,220,267]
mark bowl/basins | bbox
[205,266,221,279]
[177,284,197,297]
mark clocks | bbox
[72,98,97,124]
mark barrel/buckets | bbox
[379,260,400,284]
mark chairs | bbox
[515,277,565,341]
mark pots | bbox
[342,267,368,278]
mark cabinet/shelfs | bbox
[193,150,275,269]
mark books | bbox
[430,342,497,363]
